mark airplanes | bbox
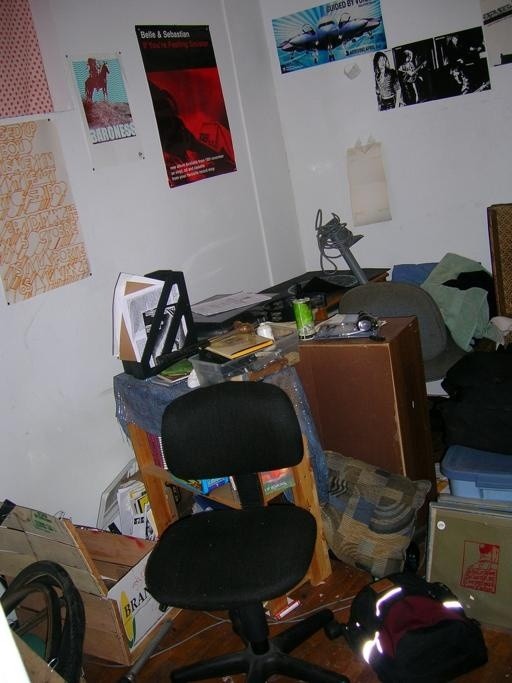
[276,11,382,64]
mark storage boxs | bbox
[435,445,511,502]
[1,498,179,671]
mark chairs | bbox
[145,380,354,683]
[337,282,477,454]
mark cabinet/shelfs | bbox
[111,335,337,619]
[240,263,390,324]
[293,312,437,482]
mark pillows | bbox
[310,446,433,581]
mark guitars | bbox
[405,61,427,82]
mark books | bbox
[94,457,159,542]
[108,269,295,385]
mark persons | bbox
[372,34,465,108]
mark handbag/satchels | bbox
[349,571,488,681]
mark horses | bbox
[85,61,110,102]
